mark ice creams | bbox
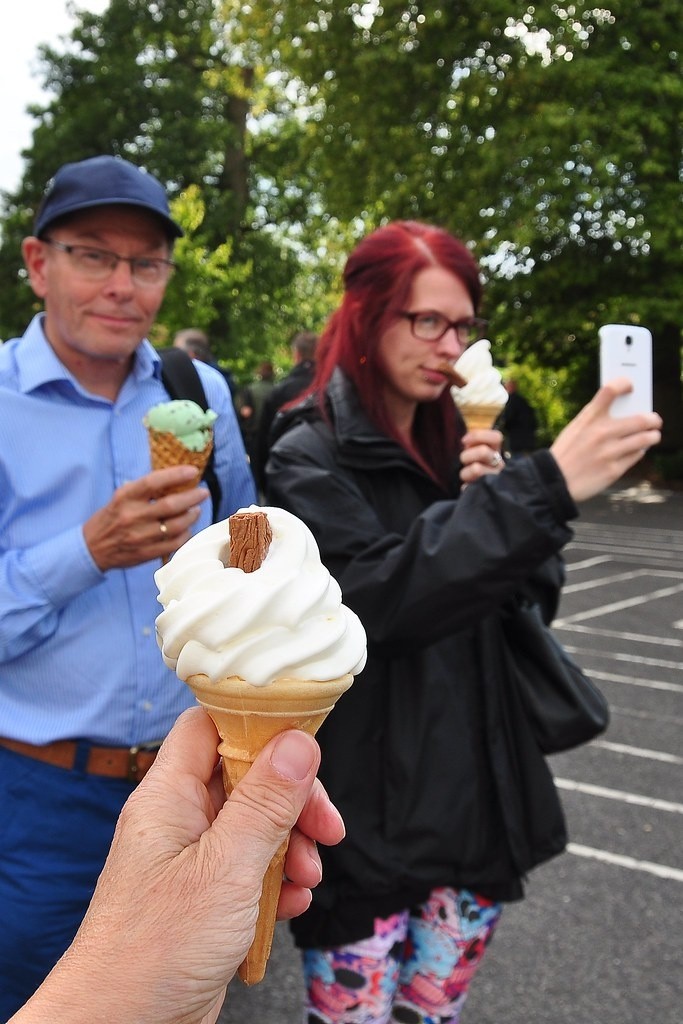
[143,398,219,564]
[155,504,368,985]
[437,337,509,435]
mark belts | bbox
[0,735,154,782]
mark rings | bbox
[489,451,503,466]
[160,519,169,541]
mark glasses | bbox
[396,308,491,349]
[38,236,181,287]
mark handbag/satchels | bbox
[503,595,610,756]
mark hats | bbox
[33,153,184,241]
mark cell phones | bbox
[598,324,652,418]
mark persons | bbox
[174,328,318,506]
[0,154,258,1023]
[7,706,346,1023]
[264,218,662,1023]
[504,381,537,460]
